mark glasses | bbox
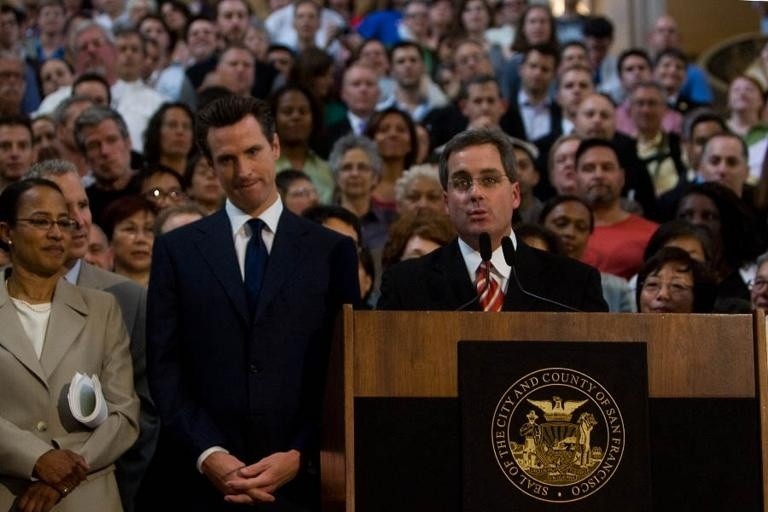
[143,188,182,199]
[447,174,508,189]
[15,217,76,235]
[640,281,692,292]
[657,28,678,33]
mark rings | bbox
[60,485,69,495]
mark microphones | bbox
[501,235,586,313]
[454,230,494,312]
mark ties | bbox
[245,219,270,290]
[475,262,507,312]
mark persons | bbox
[141,93,365,512]
[1,0,767,316]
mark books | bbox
[66,370,108,429]
